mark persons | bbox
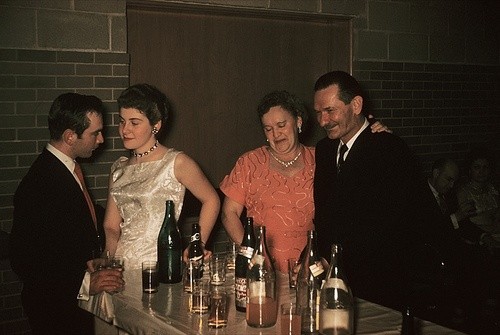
[221,91,393,276]
[299,71,411,307]
[9,93,125,335]
[411,152,500,257]
[101,85,221,271]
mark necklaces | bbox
[268,147,302,168]
[130,139,159,157]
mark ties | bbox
[73,161,100,239]
[337,143,349,177]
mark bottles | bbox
[295,230,326,335]
[318,243,355,335]
[234,216,257,312]
[399,306,416,335]
[246,225,279,328]
[185,223,204,281]
[156,200,183,284]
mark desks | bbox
[78,257,469,335]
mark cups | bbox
[91,250,126,274]
[209,254,227,286]
[207,290,227,328]
[279,303,304,335]
[287,257,304,288]
[192,279,210,314]
[142,260,159,294]
